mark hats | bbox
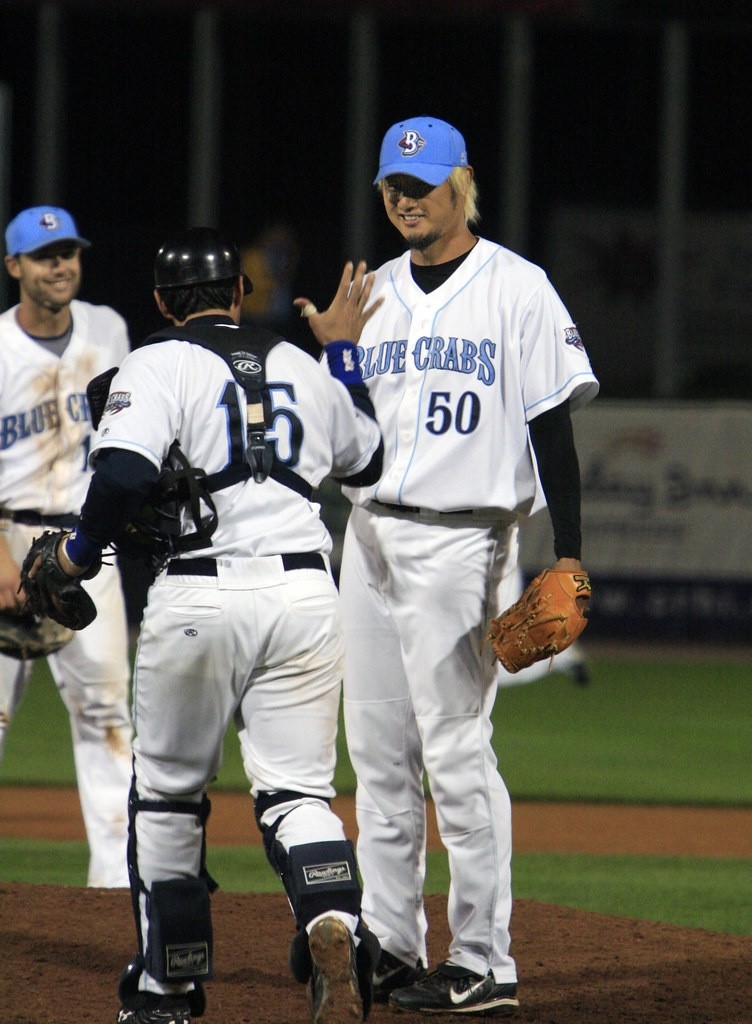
[373,116,468,186]
[5,205,92,257]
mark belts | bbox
[0,509,78,528]
[371,499,473,515]
[167,552,327,577]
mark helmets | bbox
[154,226,253,297]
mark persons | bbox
[298,116,601,1016]
[0,203,385,1024]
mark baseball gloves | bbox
[482,569,595,675]
[15,529,117,631]
[1,603,78,661]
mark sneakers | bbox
[370,951,428,1005]
[389,961,519,1020]
[309,916,364,1024]
[117,1004,192,1024]
